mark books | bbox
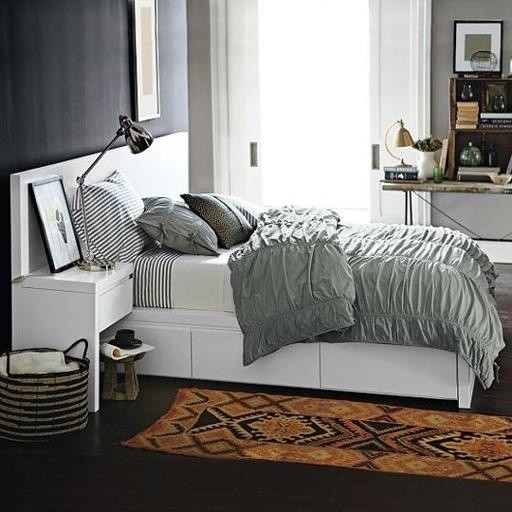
[456,101,512,129]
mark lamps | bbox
[77,110,155,271]
[381,117,420,166]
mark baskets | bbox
[0,339,88,443]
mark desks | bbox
[377,177,512,241]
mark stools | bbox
[100,351,146,403]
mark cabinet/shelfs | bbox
[445,76,512,180]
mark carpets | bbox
[121,385,511,486]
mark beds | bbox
[8,131,508,412]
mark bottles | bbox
[461,83,474,100]
[488,144,499,167]
[480,131,488,165]
[492,95,505,112]
[459,141,481,166]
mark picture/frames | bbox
[26,176,83,275]
[452,17,504,76]
[129,0,161,122]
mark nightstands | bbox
[14,259,141,416]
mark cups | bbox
[114,329,136,346]
[434,167,443,183]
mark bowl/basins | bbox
[490,174,511,185]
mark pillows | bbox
[177,186,262,249]
[70,165,147,275]
[133,194,223,259]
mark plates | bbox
[108,338,142,350]
